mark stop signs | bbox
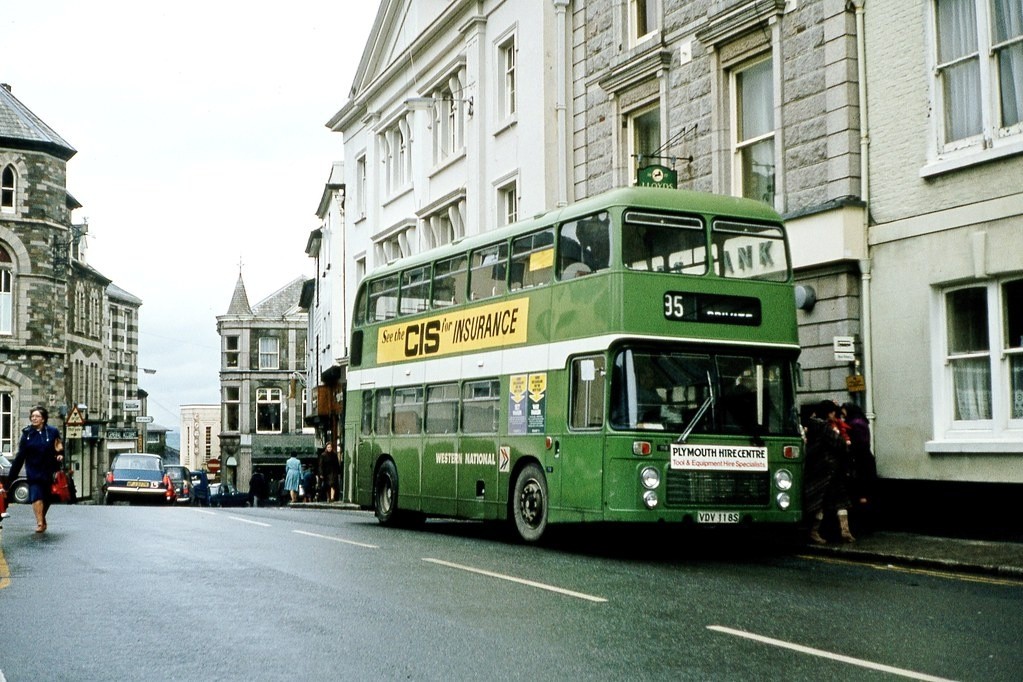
[207,459,221,471]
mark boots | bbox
[810,510,826,544]
[837,507,856,542]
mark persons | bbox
[320,442,339,503]
[250,465,267,507]
[799,401,876,544]
[302,467,319,502]
[284,451,303,503]
[9,406,64,532]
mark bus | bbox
[344,186,806,543]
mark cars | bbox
[0,452,30,503]
[163,466,252,507]
[103,453,168,505]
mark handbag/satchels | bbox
[299,485,306,497]
[51,457,77,504]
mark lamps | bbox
[404,96,474,115]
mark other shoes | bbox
[36,520,47,532]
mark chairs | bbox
[372,288,503,323]
[383,407,498,433]
